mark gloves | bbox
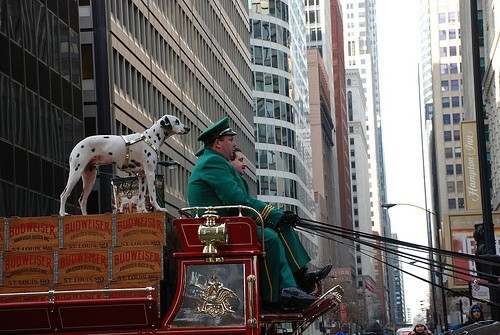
[281,211,301,229]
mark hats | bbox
[195,145,205,156]
[196,117,237,141]
[411,313,431,335]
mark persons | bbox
[228,148,332,294]
[464,304,485,326]
[187,116,319,313]
[408,314,433,335]
[194,144,208,157]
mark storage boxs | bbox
[0,211,179,319]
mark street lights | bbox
[381,203,448,332]
[409,261,439,328]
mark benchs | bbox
[162,216,263,335]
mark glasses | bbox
[472,309,480,312]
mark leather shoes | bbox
[281,285,317,306]
[260,301,294,313]
[297,264,333,295]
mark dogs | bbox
[59,115,191,216]
